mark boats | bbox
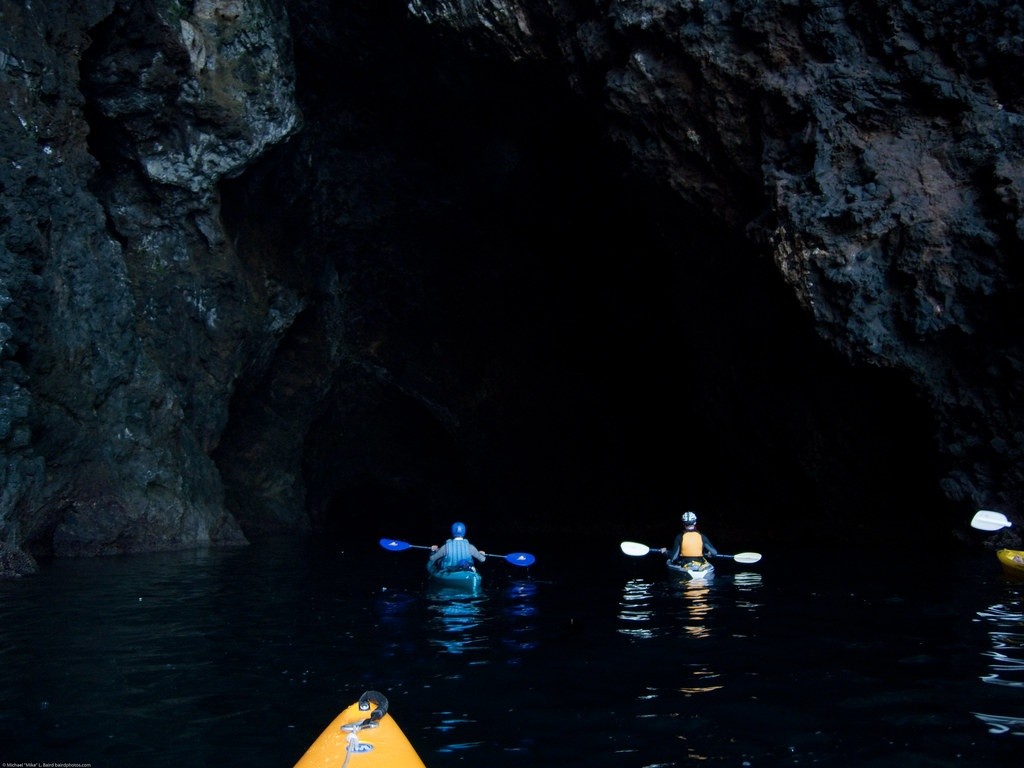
[997,548,1024,571]
[666,557,714,580]
[293,692,426,768]
[426,550,482,591]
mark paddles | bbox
[620,542,762,563]
[381,539,535,565]
[971,510,1024,530]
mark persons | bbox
[430,522,486,571]
[661,512,717,568]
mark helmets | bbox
[451,522,466,537]
[682,511,697,523]
